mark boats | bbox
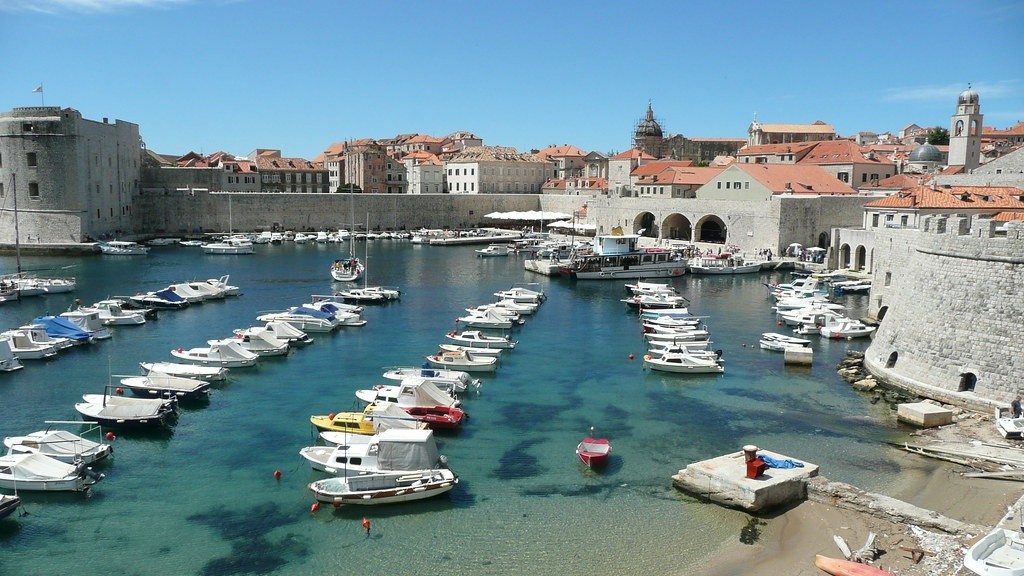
[620,280,724,374]
[299,363,481,506]
[576,436,613,466]
[0,418,113,522]
[100,241,148,255]
[760,332,813,364]
[144,238,180,245]
[256,285,401,332]
[0,268,240,371]
[558,233,764,278]
[456,280,547,329]
[762,279,877,340]
[73,318,313,428]
[475,230,592,257]
[426,330,516,372]
[181,228,486,247]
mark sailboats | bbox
[201,193,256,255]
[329,152,365,283]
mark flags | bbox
[32,85,42,93]
